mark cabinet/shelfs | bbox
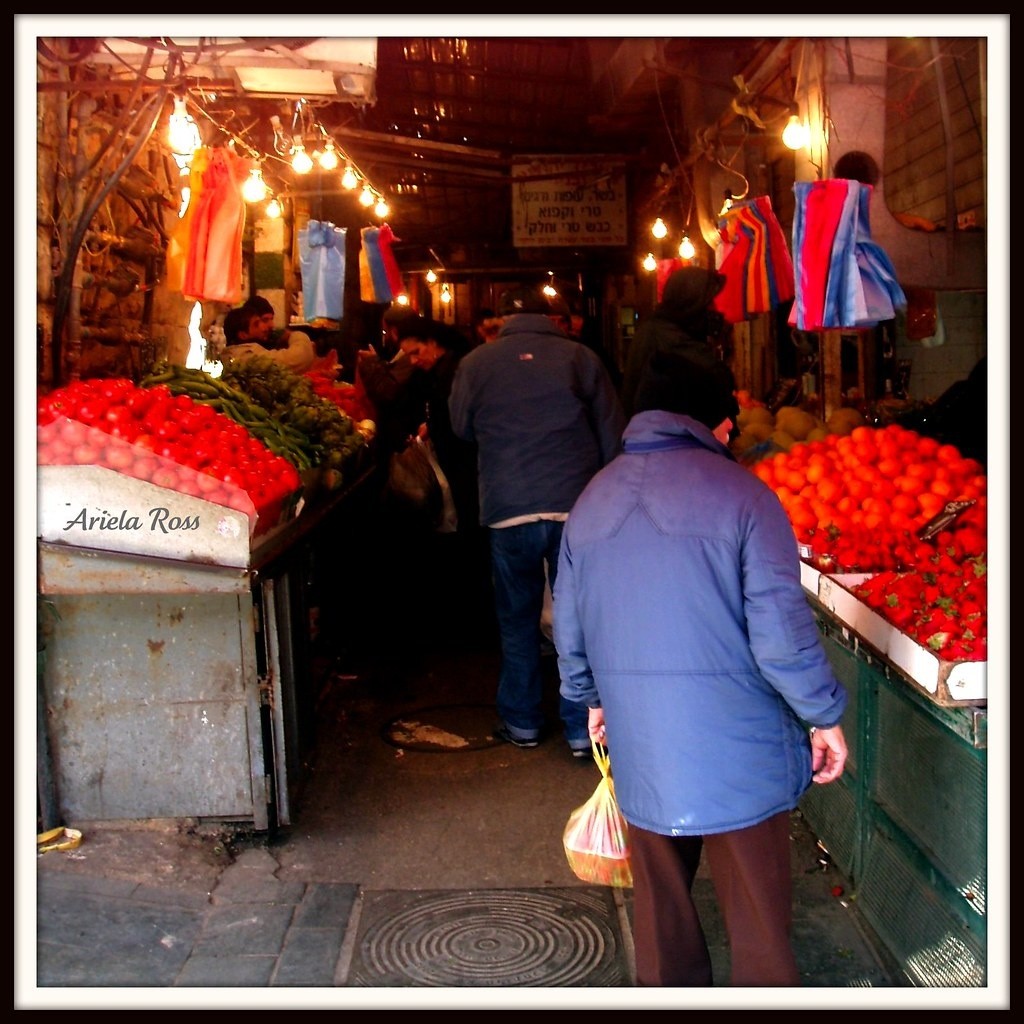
[37,540,299,831]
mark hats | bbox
[656,269,725,320]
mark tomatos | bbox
[34,377,300,533]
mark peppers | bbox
[793,525,989,662]
[138,362,327,473]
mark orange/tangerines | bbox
[752,425,986,533]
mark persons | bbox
[220,266,741,762]
[551,350,846,987]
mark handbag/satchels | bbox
[416,434,458,532]
[388,442,433,502]
[560,727,633,890]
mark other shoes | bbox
[491,719,539,748]
[572,746,592,757]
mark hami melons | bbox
[728,404,862,462]
